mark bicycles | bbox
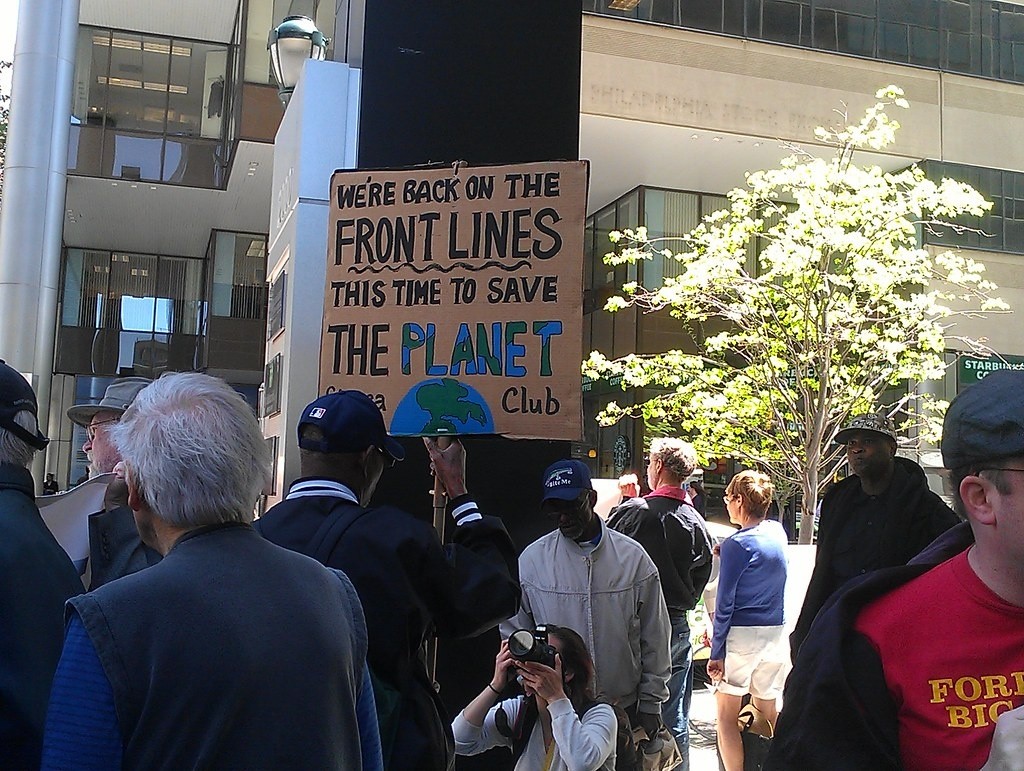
[688,645,754,742]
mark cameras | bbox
[508,624,558,680]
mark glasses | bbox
[645,460,658,466]
[86,417,121,442]
[723,495,739,505]
[975,468,1024,477]
[363,444,396,470]
[546,491,591,515]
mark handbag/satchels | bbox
[716,711,773,771]
[633,725,683,771]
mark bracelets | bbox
[488,683,501,695]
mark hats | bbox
[833,413,897,445]
[0,360,50,451]
[541,460,592,505]
[941,368,1024,467]
[65,377,153,429]
[297,390,406,461]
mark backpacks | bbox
[576,699,636,771]
[245,503,404,771]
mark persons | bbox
[762,369,1024,770]
[707,470,789,771]
[790,413,964,666]
[39,371,387,771]
[0,358,718,771]
